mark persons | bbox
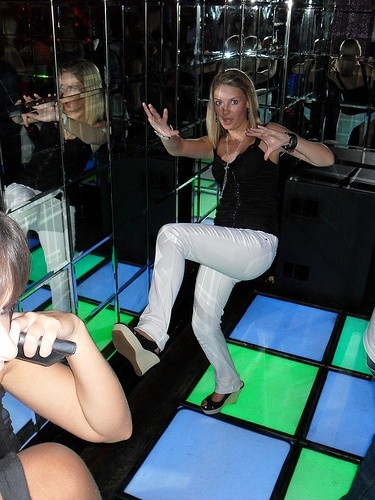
[0,212,133,500]
[328,38,375,149]
[112,69,334,414]
[4,60,109,313]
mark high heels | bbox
[199,380,244,416]
[111,321,164,379]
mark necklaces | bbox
[64,131,73,142]
[216,133,250,199]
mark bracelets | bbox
[152,125,173,140]
[283,133,297,152]
[63,113,67,126]
